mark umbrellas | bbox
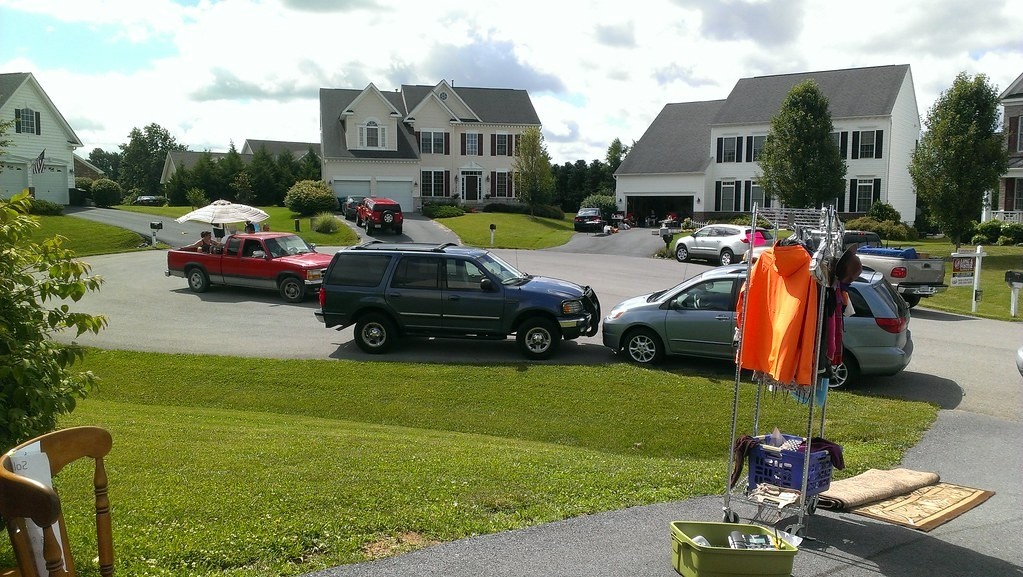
[174,199,270,250]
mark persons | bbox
[244,221,255,233]
[645,210,657,226]
[211,223,225,241]
[668,214,673,220]
[604,224,612,235]
[623,217,635,228]
[192,231,220,253]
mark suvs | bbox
[674,224,773,267]
[341,194,366,221]
[353,195,404,236]
[603,258,914,392]
[314,241,602,360]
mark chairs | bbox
[1,426,114,577]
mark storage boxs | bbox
[749,433,835,496]
[668,519,798,577]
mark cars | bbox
[132,195,160,207]
[574,207,609,233]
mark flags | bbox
[33,151,44,173]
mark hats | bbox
[835,243,862,284]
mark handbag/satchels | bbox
[809,207,835,287]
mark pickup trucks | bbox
[165,232,337,305]
[741,227,949,308]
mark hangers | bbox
[783,221,844,257]
